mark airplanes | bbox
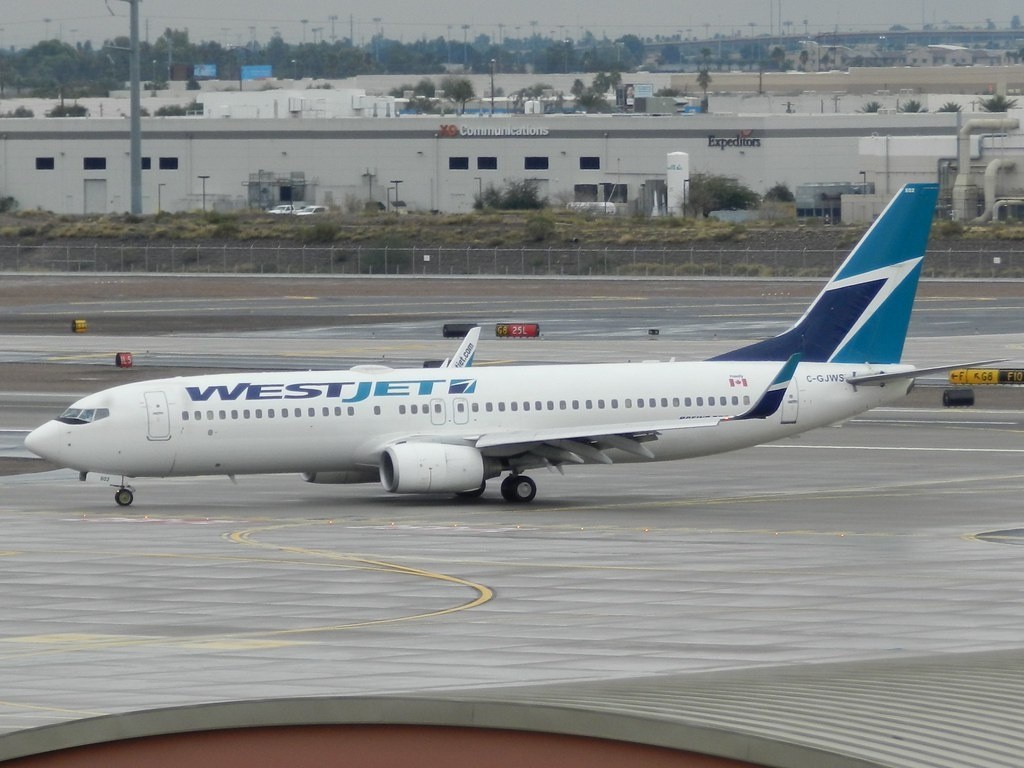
[25,182,1012,502]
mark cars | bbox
[268,205,296,215]
[296,205,330,215]
